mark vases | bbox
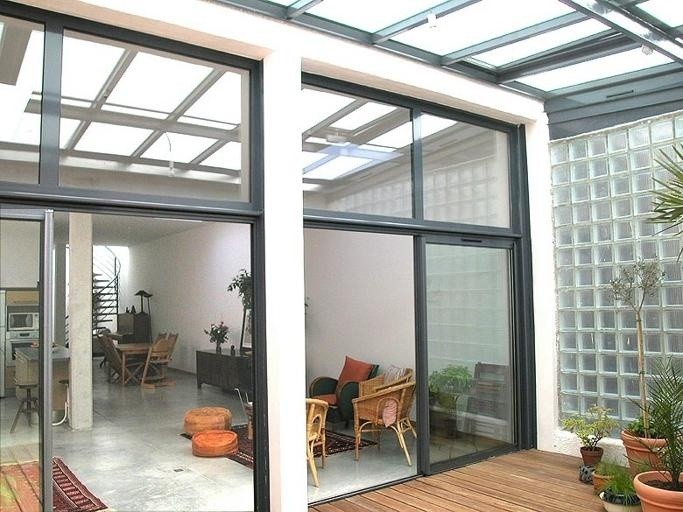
[215,346,221,353]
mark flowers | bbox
[203,321,229,345]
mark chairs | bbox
[92,327,178,388]
[307,356,417,488]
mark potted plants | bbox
[561,255,683,512]
[427,364,475,440]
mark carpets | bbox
[0,458,108,512]
[180,425,378,470]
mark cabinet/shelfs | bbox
[195,349,253,403]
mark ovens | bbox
[7,332,39,366]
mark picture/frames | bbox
[240,306,253,351]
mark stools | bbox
[184,406,233,437]
[9,380,41,433]
[192,429,238,458]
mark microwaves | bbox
[8,312,39,330]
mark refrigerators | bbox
[0,291,6,398]
